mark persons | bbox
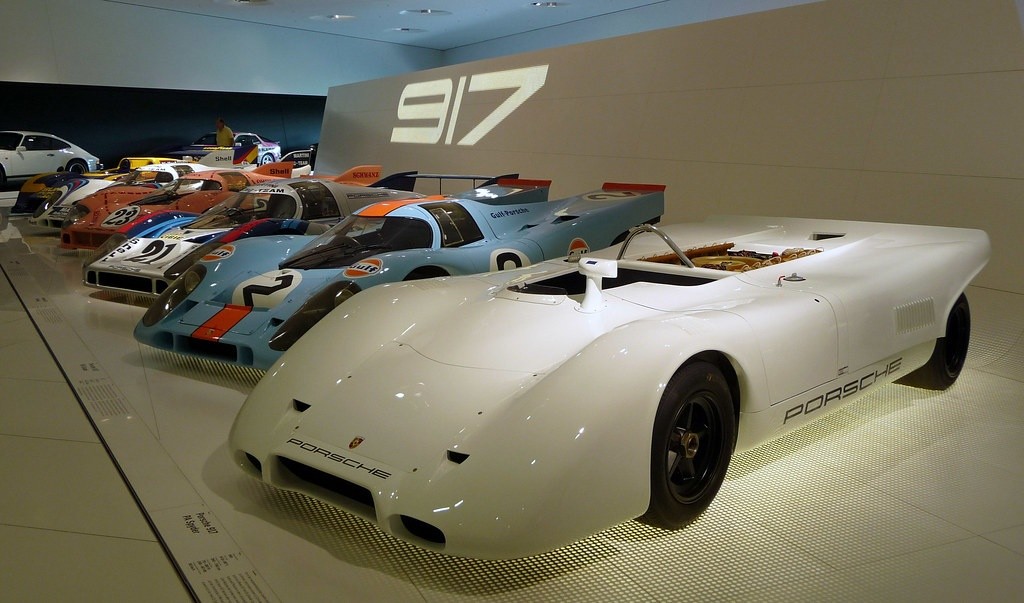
[214,117,235,146]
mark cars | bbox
[0,129,103,191]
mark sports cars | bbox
[12,131,991,562]
